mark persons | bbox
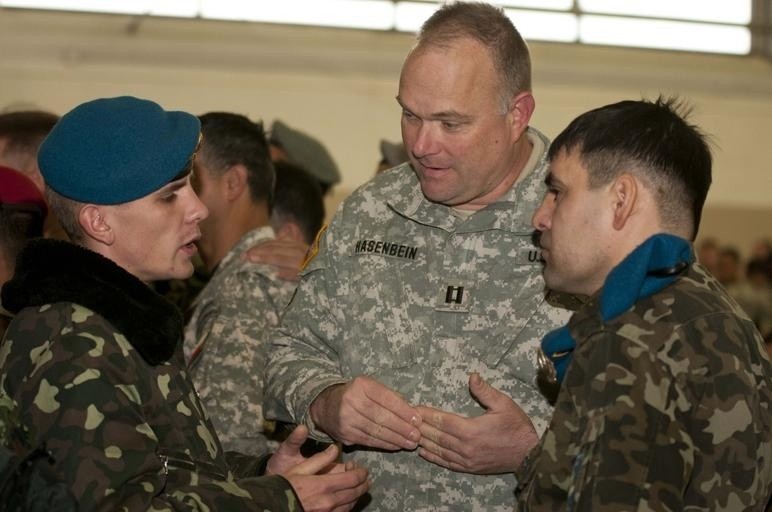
[1,95,413,509]
[512,97,771,511]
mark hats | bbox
[36,94,203,204]
[0,166,48,220]
[269,120,341,186]
[535,233,696,383]
[381,140,409,167]
